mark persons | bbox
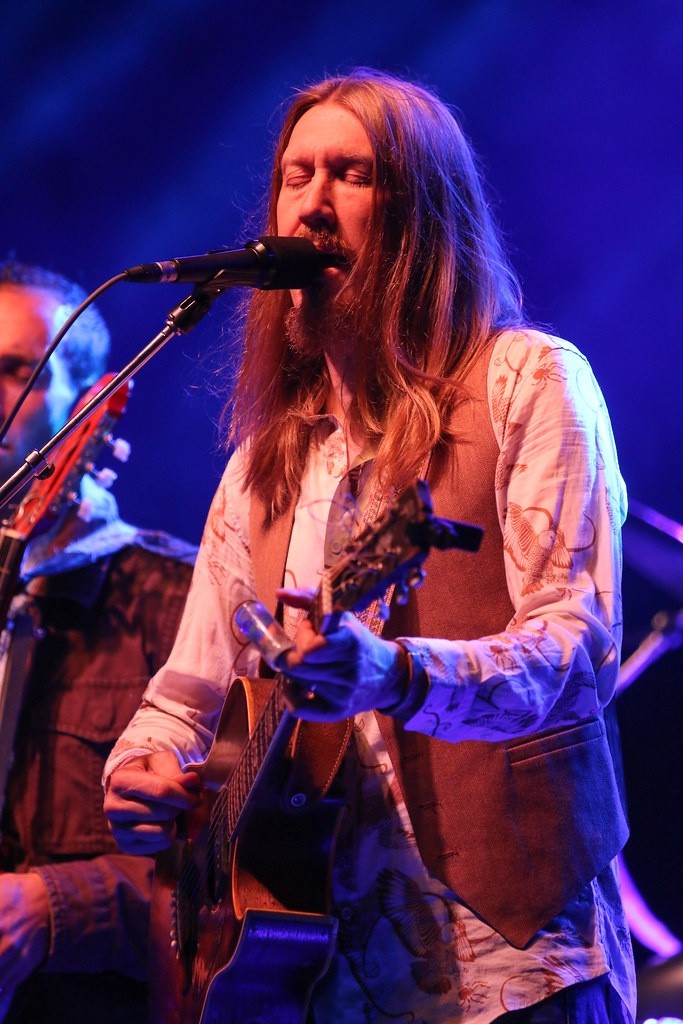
[0,258,202,1024]
[100,68,641,1024]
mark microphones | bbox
[123,240,322,289]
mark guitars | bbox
[136,479,484,1023]
